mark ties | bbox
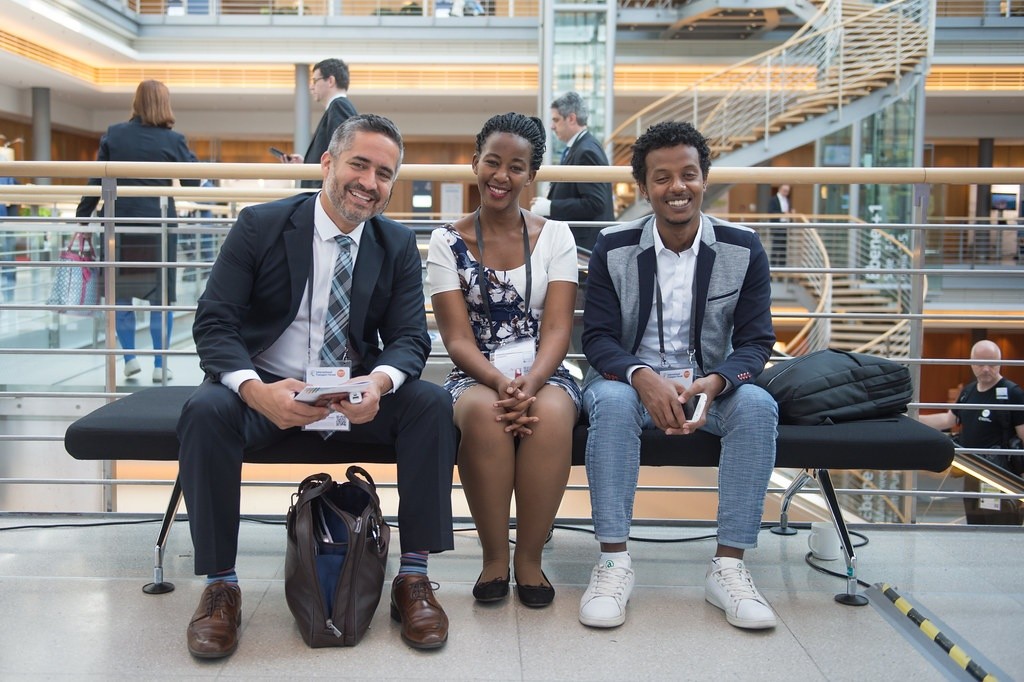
[321,235,356,368]
[548,147,569,186]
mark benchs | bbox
[65,385,955,608]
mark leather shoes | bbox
[188,583,242,657]
[391,573,449,649]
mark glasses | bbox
[311,77,323,85]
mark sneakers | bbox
[152,366,172,382]
[704,557,776,628]
[579,561,635,627]
[124,358,141,377]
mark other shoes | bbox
[514,568,555,606]
[472,565,511,603]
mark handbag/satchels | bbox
[45,233,102,316]
[285,465,390,648]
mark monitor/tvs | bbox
[990,192,1017,210]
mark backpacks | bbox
[757,347,914,425]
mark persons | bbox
[76,81,201,382]
[0,177,16,303]
[900,339,1024,525]
[280,57,359,195]
[425,112,586,608]
[770,183,796,282]
[179,179,216,280]
[578,122,780,630]
[175,113,456,660]
[530,92,615,379]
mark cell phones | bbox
[269,147,291,161]
[681,392,708,422]
